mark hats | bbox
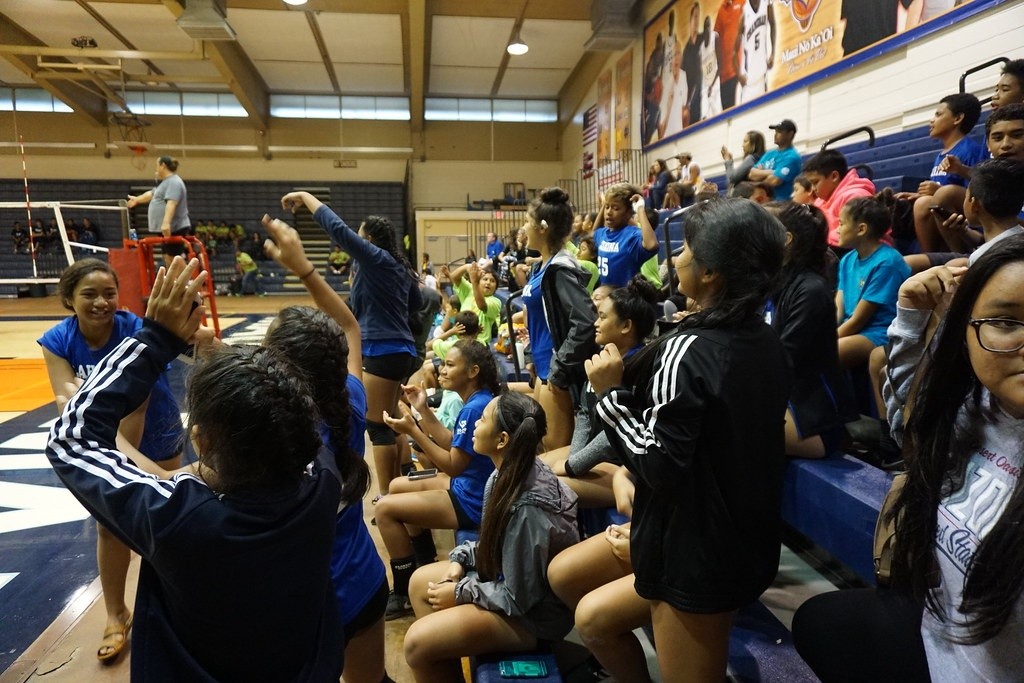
[769,119,797,132]
[674,151,693,159]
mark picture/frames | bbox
[642,0,1011,156]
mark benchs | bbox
[472,200,529,210]
[430,110,997,683]
[0,181,408,298]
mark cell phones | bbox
[178,295,204,364]
[500,660,548,677]
[407,468,438,480]
[928,206,963,224]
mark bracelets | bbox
[299,268,317,279]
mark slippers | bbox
[97,613,133,659]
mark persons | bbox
[10,58,1024,682]
[642,1,964,145]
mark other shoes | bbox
[385,588,415,621]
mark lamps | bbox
[506,29,529,56]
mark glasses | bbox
[968,316,1023,354]
